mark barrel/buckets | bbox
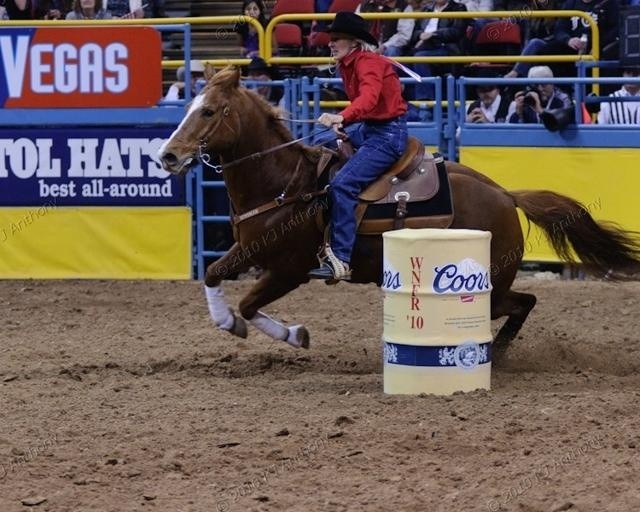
[382,228,494,396]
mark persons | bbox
[311,6,409,283]
[0,1,640,126]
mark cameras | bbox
[523,89,542,106]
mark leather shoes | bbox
[308,267,359,280]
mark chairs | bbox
[270,0,522,70]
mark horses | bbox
[156,61,640,370]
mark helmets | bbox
[311,11,379,48]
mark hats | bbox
[619,57,640,70]
[526,66,553,78]
[176,61,205,82]
